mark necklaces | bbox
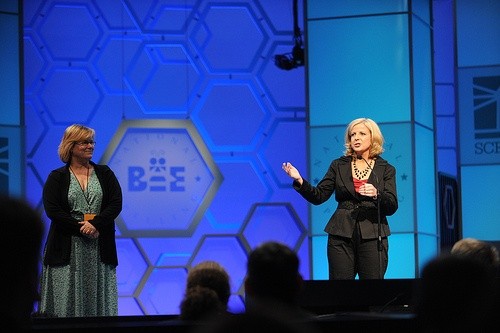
[353,159,372,180]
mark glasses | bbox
[74,141,96,146]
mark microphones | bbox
[357,155,379,194]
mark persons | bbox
[281,118,398,280]
[41,123,123,317]
[179,240,305,323]
[413,236,500,333]
